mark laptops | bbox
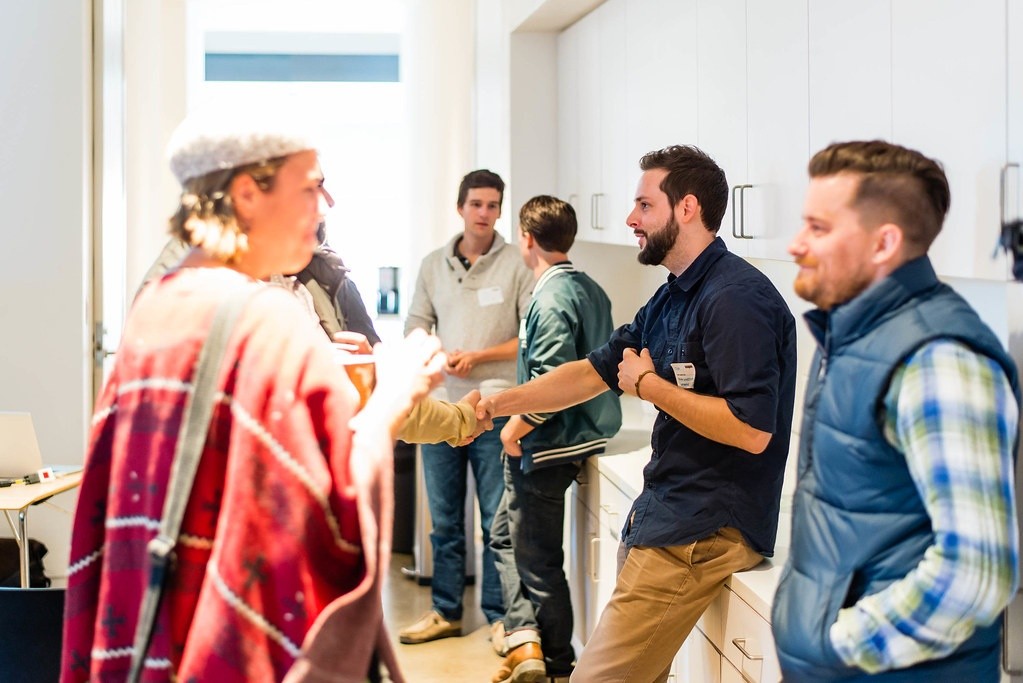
[0,412,85,477]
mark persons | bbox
[494,195,623,683]
[458,145,797,683]
[770,140,1023,683]
[400,168,538,657]
[58,118,494,683]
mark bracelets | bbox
[635,369,657,400]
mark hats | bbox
[167,95,319,177]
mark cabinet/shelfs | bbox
[555,0,1023,281]
[563,461,785,683]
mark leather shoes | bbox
[492,642,546,683]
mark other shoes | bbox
[399,610,463,644]
[489,621,506,656]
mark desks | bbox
[0,470,85,588]
